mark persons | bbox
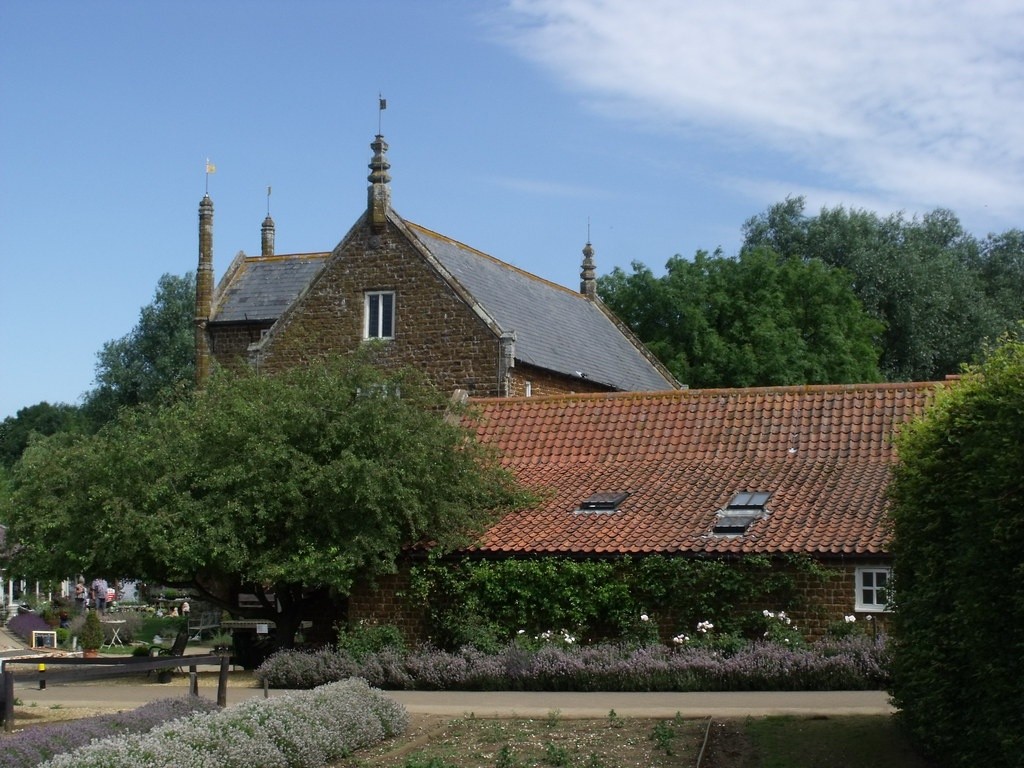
[73,575,86,614]
[105,581,116,614]
[91,578,108,618]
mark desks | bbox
[99,620,126,650]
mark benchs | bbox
[186,610,221,641]
[1,651,230,734]
